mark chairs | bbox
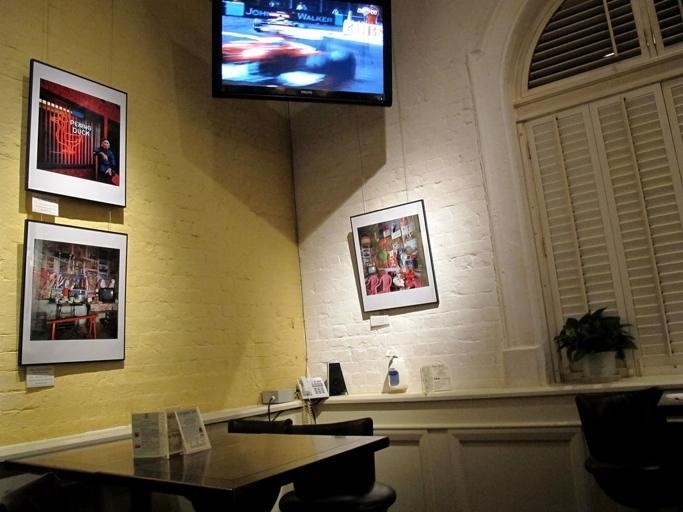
[277,422,397,511]
[185,417,288,511]
[568,384,683,511]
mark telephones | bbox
[295,376,330,401]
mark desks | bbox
[4,424,389,511]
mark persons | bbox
[94,137,118,186]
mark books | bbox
[131,405,212,459]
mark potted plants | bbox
[551,306,641,380]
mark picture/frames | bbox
[19,219,132,368]
[345,199,443,317]
[24,51,129,209]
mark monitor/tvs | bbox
[212,0,391,107]
